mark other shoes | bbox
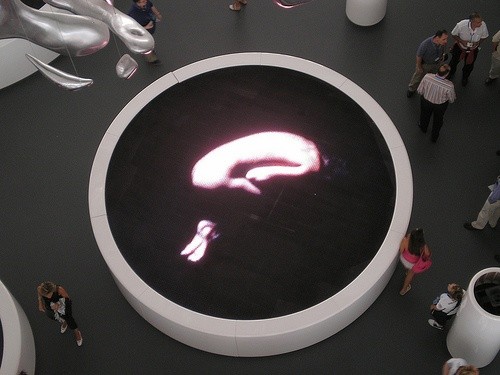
[418,122,421,127]
[486,77,497,85]
[462,76,468,85]
[152,61,160,65]
[60,324,68,334]
[407,90,415,97]
[400,284,411,295]
[429,319,444,329]
[464,222,482,230]
[230,4,241,11]
[76,331,83,346]
[483,224,494,231]
[237,0,247,5]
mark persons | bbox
[399,227,430,294]
[464,175,500,230]
[417,64,456,143]
[443,358,479,375]
[485,30,500,84]
[229,0,246,11]
[37,281,82,346]
[450,10,489,85]
[407,28,448,98]
[428,284,465,329]
[128,0,162,65]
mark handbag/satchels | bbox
[410,253,432,274]
[433,309,447,325]
